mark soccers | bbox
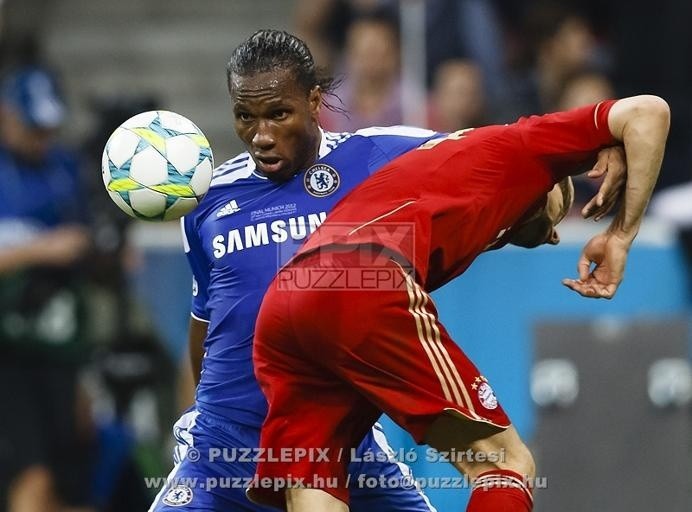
[101,110,214,222]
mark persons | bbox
[1,34,171,512]
[300,1,618,132]
[146,29,627,512]
[246,94,673,512]
